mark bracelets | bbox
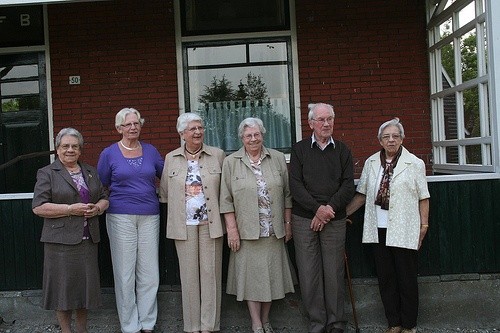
[68,206,72,217]
[285,222,292,223]
[98,210,104,215]
[421,224,428,227]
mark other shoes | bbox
[263,321,275,333]
[251,325,264,333]
[401,326,418,333]
[385,326,401,333]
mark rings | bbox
[319,224,320,225]
[327,219,330,221]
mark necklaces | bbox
[121,140,141,150]
[247,152,261,162]
[185,145,202,158]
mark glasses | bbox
[120,122,141,128]
[59,145,80,150]
[311,118,334,123]
[185,126,204,132]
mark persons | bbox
[346,119,430,333]
[158,113,227,333]
[288,103,355,333]
[97,108,164,333]
[220,118,298,333]
[31,128,110,333]
